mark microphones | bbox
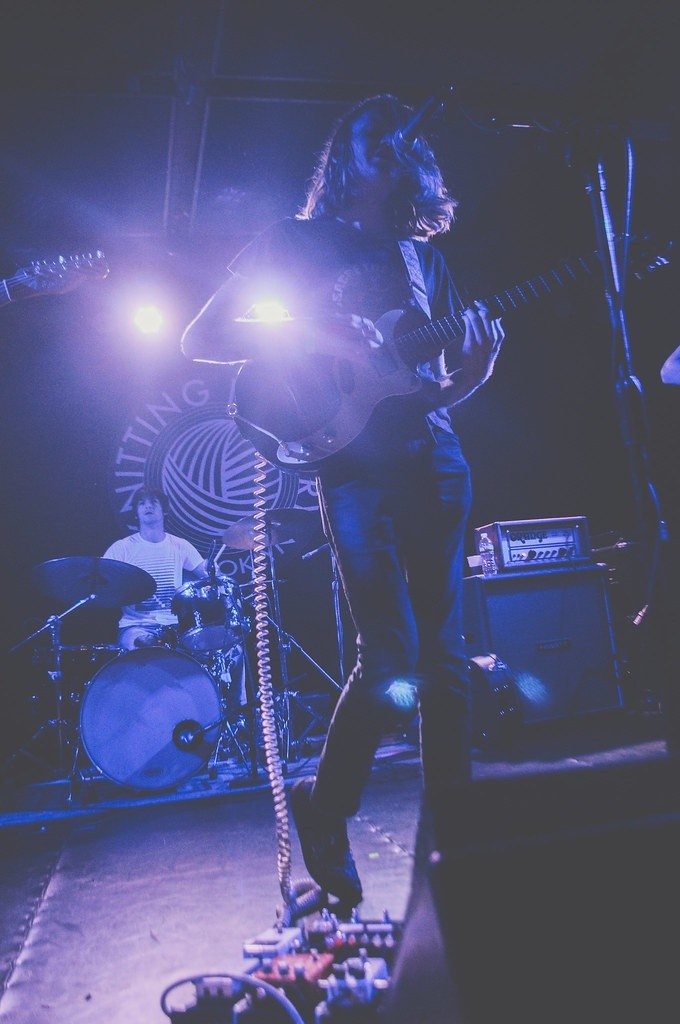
[392,83,449,153]
[177,723,215,746]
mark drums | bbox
[169,574,252,654]
[77,642,227,795]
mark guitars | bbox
[226,224,677,481]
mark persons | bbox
[100,485,222,650]
[660,344,680,384]
[180,93,506,905]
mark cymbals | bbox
[221,506,314,552]
[27,554,158,611]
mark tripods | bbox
[0,590,97,783]
[265,519,331,777]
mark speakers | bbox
[461,562,624,730]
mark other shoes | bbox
[290,776,362,901]
